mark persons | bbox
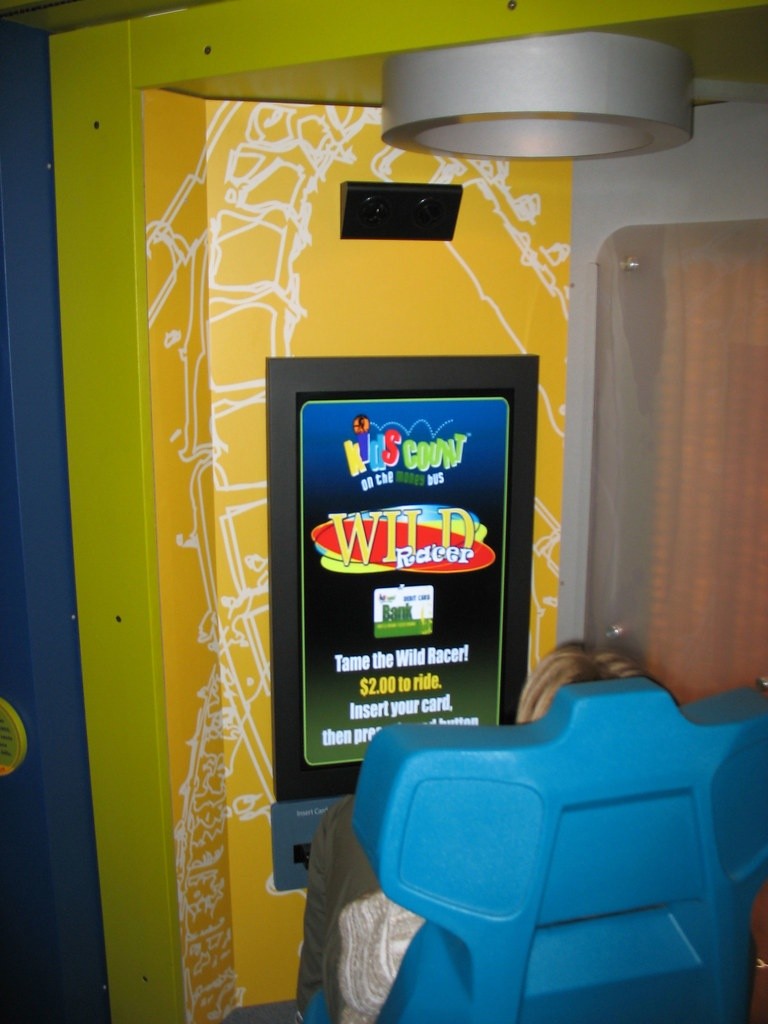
[295,639,680,1024]
